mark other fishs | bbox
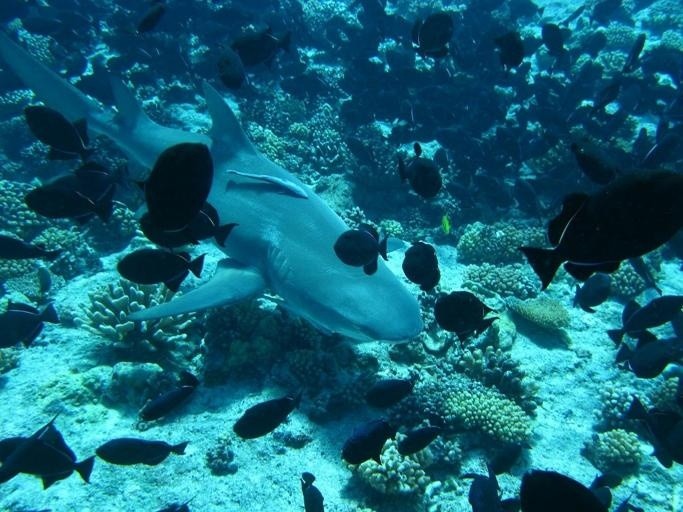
[0,0,683,512]
